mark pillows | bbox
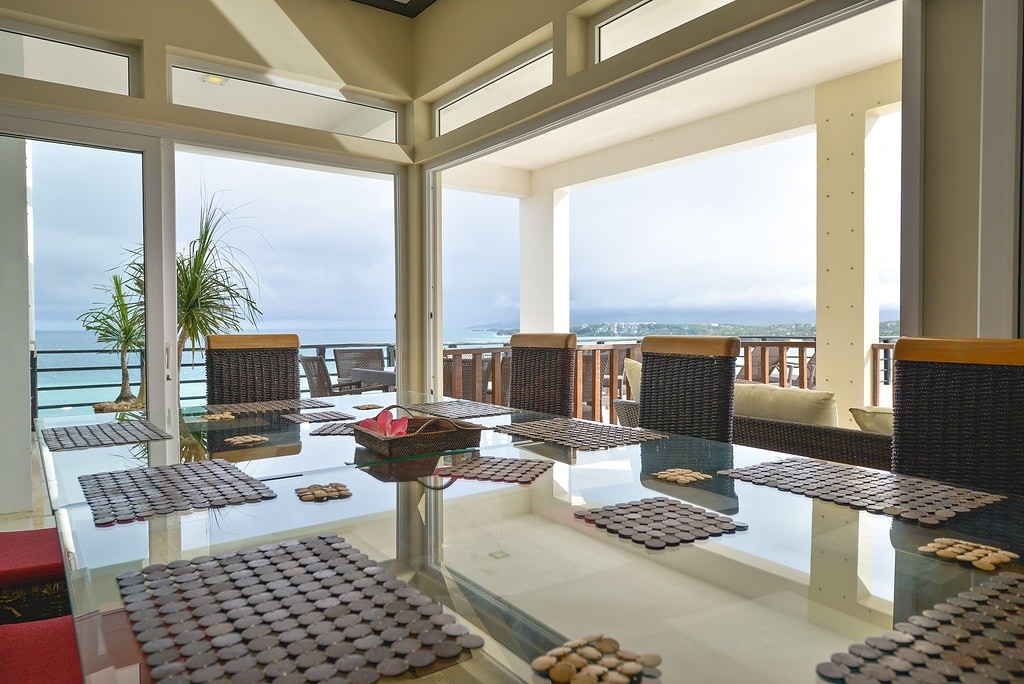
[732,383,838,427]
[624,357,643,403]
[847,406,894,436]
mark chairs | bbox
[508,333,577,418]
[333,348,385,392]
[637,335,741,444]
[203,333,301,405]
[298,354,390,399]
[890,336,1024,496]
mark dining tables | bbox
[32,389,1024,684]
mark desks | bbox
[349,366,396,395]
